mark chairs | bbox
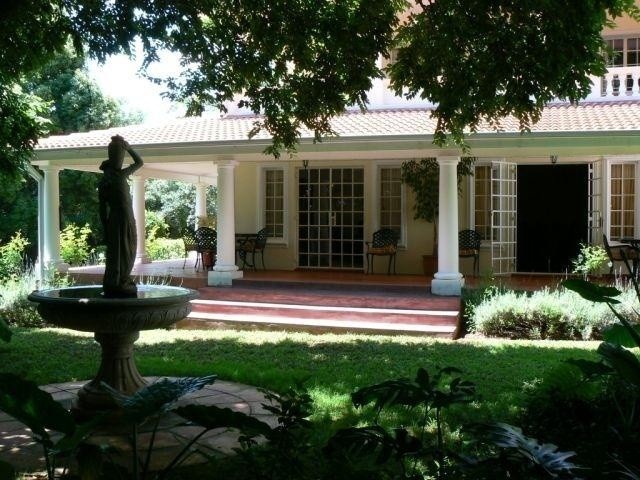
[181,225,271,273]
[365,227,399,276]
[602,233,640,280]
[458,229,481,278]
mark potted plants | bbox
[399,157,478,277]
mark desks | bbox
[620,238,640,280]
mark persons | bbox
[97,134,145,297]
